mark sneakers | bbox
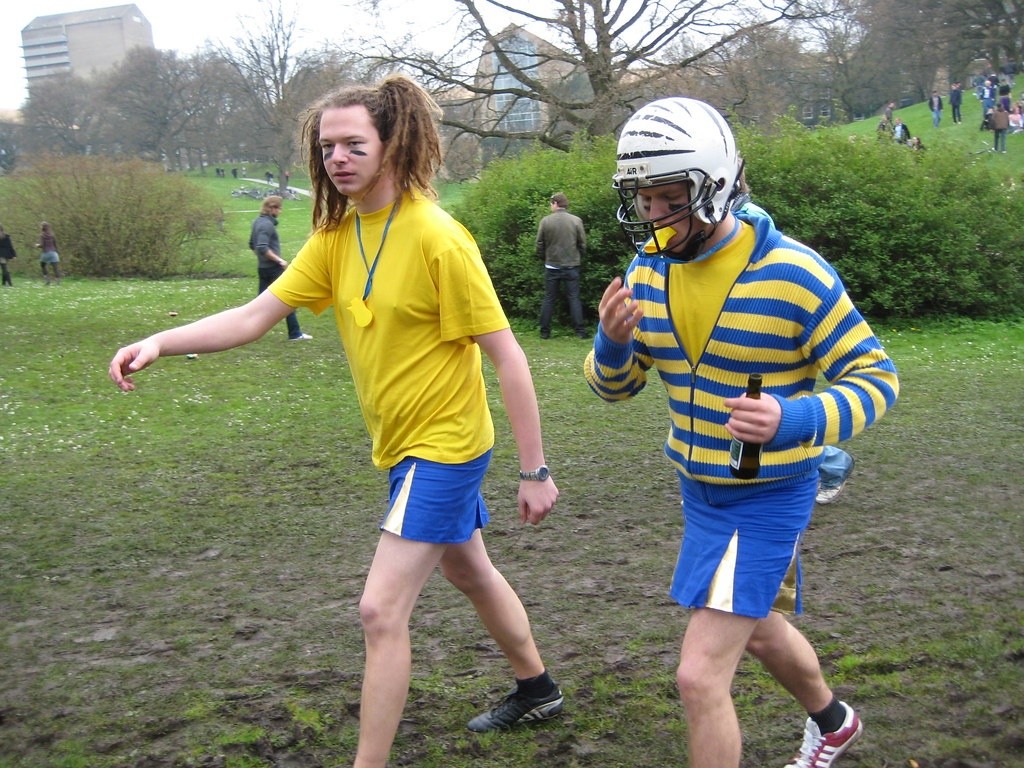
[816,455,855,504]
[466,683,564,732]
[289,333,313,340]
[784,701,864,768]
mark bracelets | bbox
[278,258,283,264]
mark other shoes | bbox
[540,334,549,339]
[577,333,591,338]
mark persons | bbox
[248,195,313,340]
[232,168,237,177]
[739,169,855,506]
[216,208,225,230]
[535,194,591,338]
[875,103,927,151]
[583,98,899,767]
[948,81,964,125]
[991,103,1009,154]
[285,170,289,181]
[0,226,17,286]
[971,57,1024,135]
[31,222,62,285]
[265,171,273,184]
[929,91,943,127]
[216,168,225,177]
[241,167,247,177]
[108,79,564,768]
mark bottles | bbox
[729,374,764,479]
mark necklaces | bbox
[346,200,398,329]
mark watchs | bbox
[519,465,549,482]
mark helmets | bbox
[611,98,745,263]
